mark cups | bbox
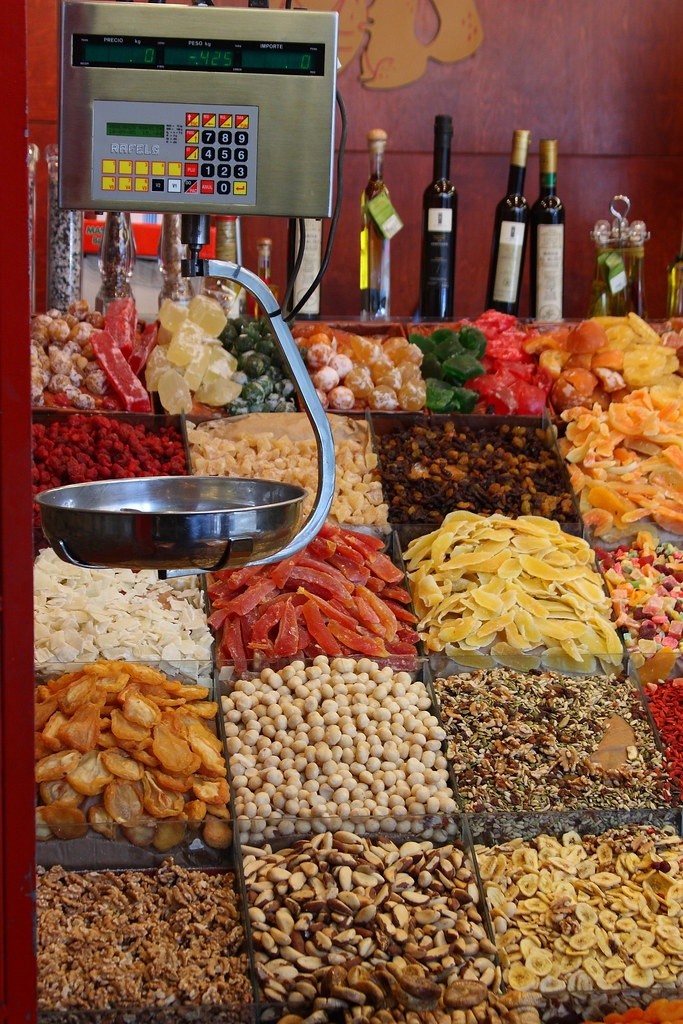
[588,241,644,324]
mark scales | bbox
[32,0,346,580]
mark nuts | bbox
[37,854,257,1024]
[239,831,545,1024]
[430,668,683,838]
[471,830,683,1024]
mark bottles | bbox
[420,115,457,323]
[360,130,393,322]
[484,130,531,318]
[205,217,247,320]
[288,217,322,320]
[252,239,279,321]
[530,139,564,321]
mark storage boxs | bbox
[30,408,683,1024]
[155,319,293,432]
[294,321,431,416]
[30,313,155,432]
[524,322,581,438]
[406,322,557,424]
